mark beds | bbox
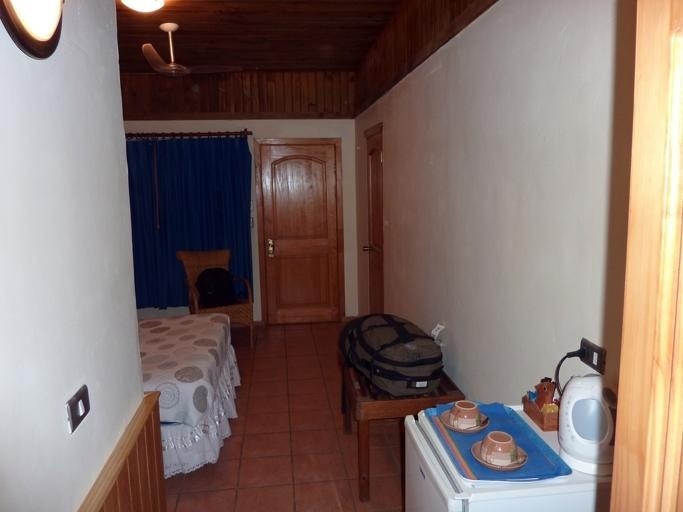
[136,313,242,478]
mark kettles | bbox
[558,372,614,475]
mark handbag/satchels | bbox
[195,268,235,306]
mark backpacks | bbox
[341,313,444,397]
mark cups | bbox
[448,400,481,429]
[480,430,518,466]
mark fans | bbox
[130,22,243,79]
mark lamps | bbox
[0,0,65,59]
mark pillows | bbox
[195,267,240,305]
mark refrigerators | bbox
[404,404,612,511]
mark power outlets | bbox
[580,338,606,375]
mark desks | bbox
[339,315,466,502]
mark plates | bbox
[471,440,528,472]
[440,409,489,433]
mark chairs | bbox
[176,248,255,359]
